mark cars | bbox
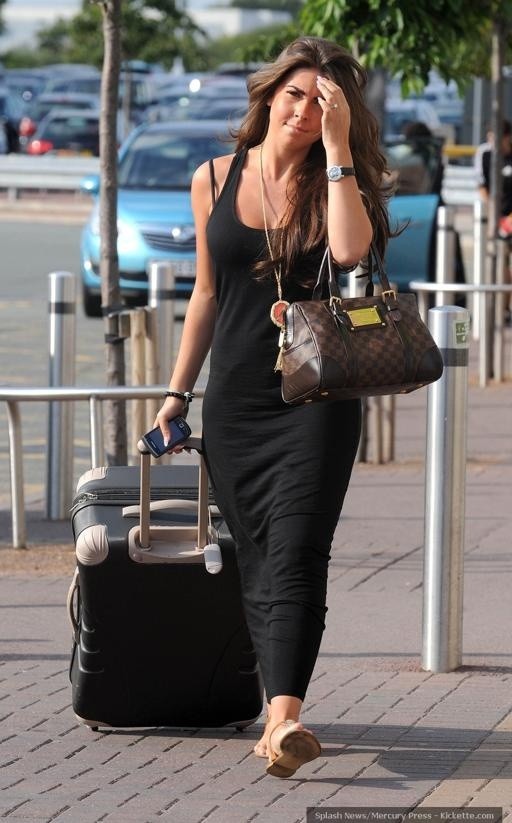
[70,114,444,322]
[1,60,511,193]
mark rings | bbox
[330,103,338,109]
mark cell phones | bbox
[141,416,192,458]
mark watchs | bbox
[324,163,355,182]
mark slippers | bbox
[255,720,321,779]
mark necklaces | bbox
[258,139,295,330]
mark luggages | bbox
[67,437,264,731]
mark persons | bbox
[384,116,512,325]
[143,34,396,781]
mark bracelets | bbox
[162,386,195,403]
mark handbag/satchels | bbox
[276,290,443,408]
